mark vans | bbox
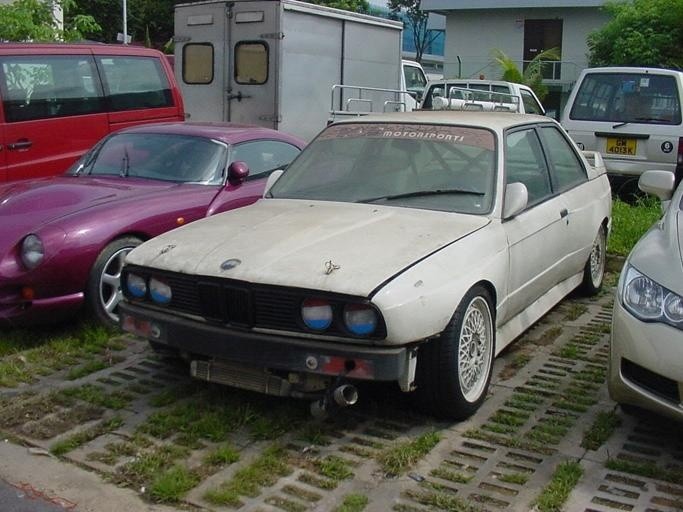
[561,66,683,196]
[0,43,184,185]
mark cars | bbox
[573,97,619,121]
[0,122,347,333]
[106,55,174,92]
[118,110,612,420]
[607,170,683,423]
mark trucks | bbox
[173,0,428,155]
[326,73,546,127]
